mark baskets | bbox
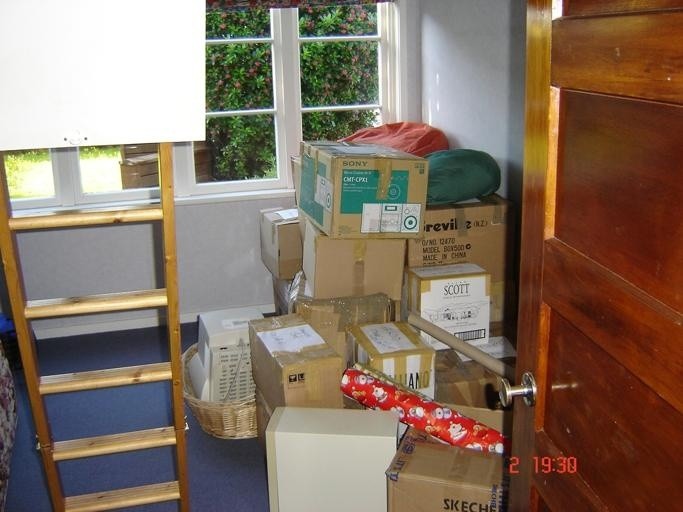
[182,344,258,440]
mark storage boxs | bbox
[197,307,265,405]
[249,142,516,508]
[119,145,213,190]
